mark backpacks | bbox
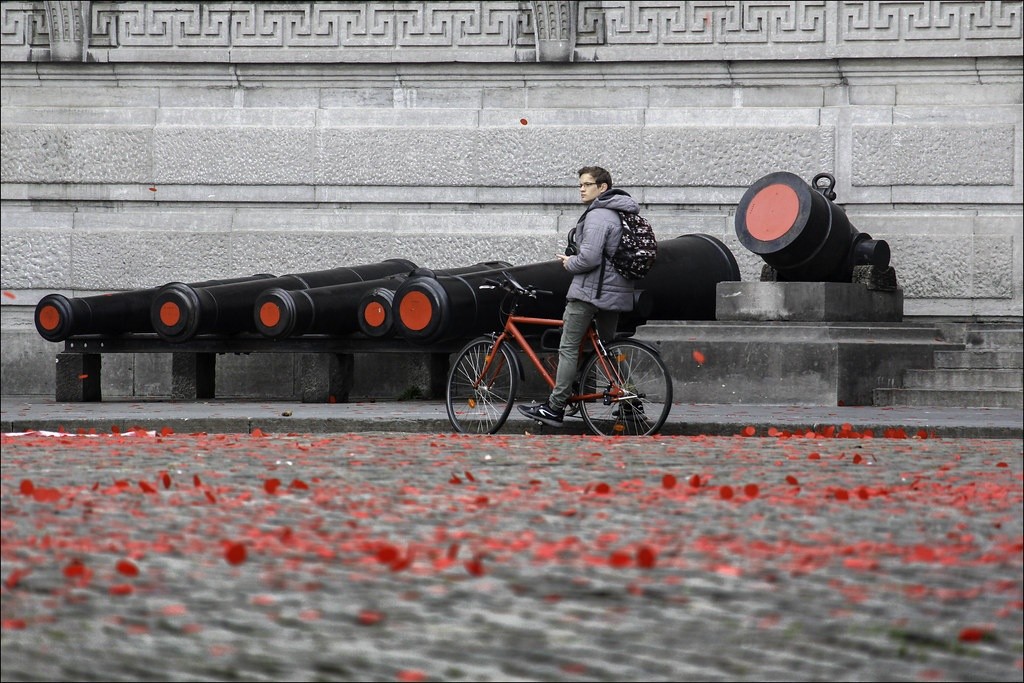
[594,209,657,300]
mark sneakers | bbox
[517,403,564,427]
[612,402,647,421]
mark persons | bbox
[516,166,647,427]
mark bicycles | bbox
[445,269,674,437]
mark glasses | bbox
[577,182,598,187]
